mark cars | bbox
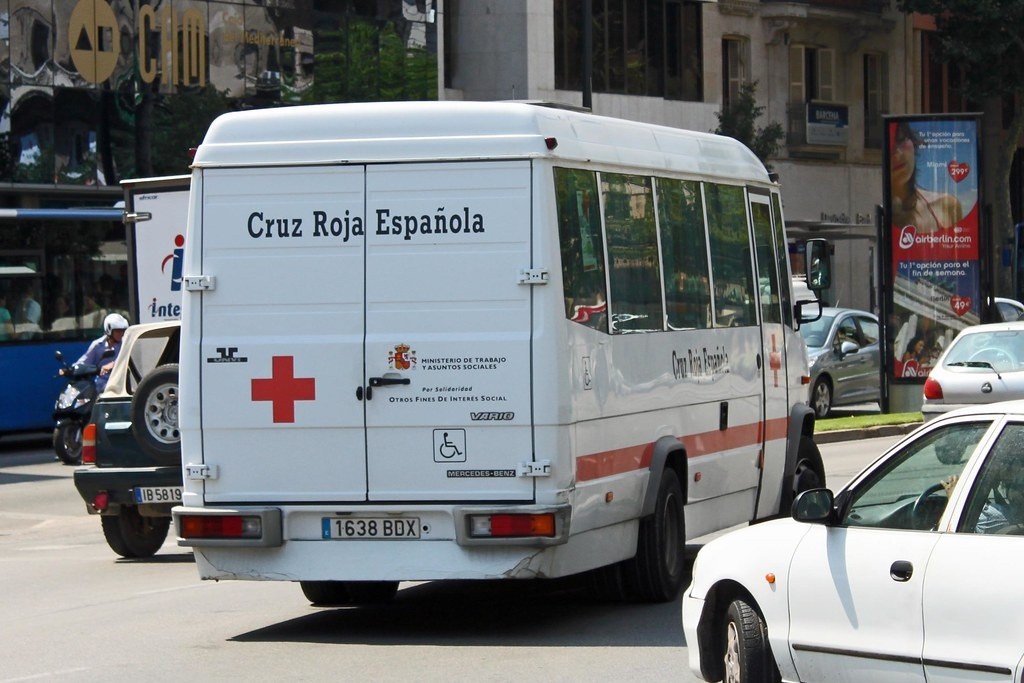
[986,296,1024,324]
[796,307,886,420]
[920,321,1024,466]
[681,399,1023,683]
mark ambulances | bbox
[168,99,836,609]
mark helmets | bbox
[104,313,129,337]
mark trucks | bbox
[118,172,193,395]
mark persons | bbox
[889,121,963,234]
[0,275,125,340]
[901,337,932,376]
[939,462,1024,535]
[59,313,130,392]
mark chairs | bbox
[995,466,1024,535]
[0,308,130,334]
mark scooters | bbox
[49,346,116,466]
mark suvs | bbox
[72,320,183,559]
